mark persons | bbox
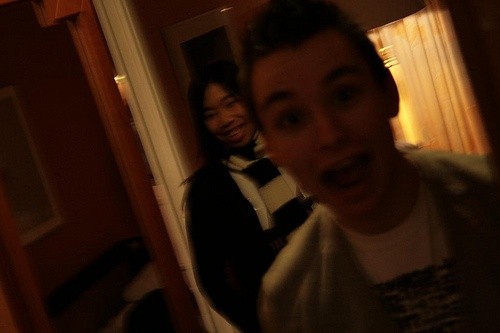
[242,0,500,333]
[185,70,320,332]
